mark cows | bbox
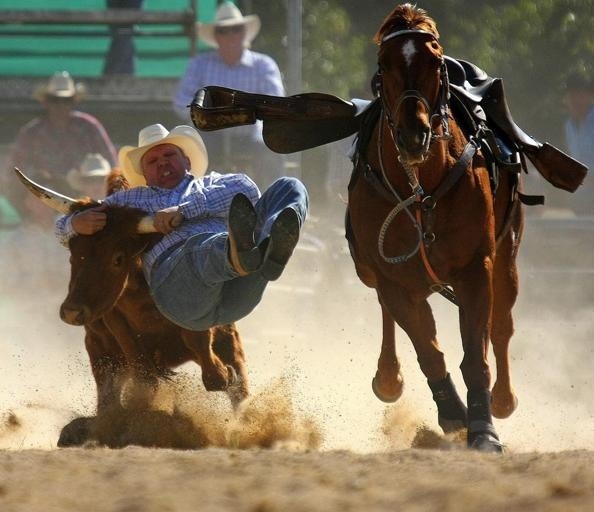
[13,166,251,448]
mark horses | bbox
[348,4,527,455]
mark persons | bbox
[562,76,593,208]
[55,144,117,280]
[326,124,357,219]
[167,1,295,175]
[0,169,74,275]
[0,67,116,212]
[53,119,310,333]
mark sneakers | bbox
[261,207,299,280]
[228,193,261,275]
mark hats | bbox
[196,1,261,48]
[67,154,120,193]
[119,124,209,188]
[30,70,85,103]
